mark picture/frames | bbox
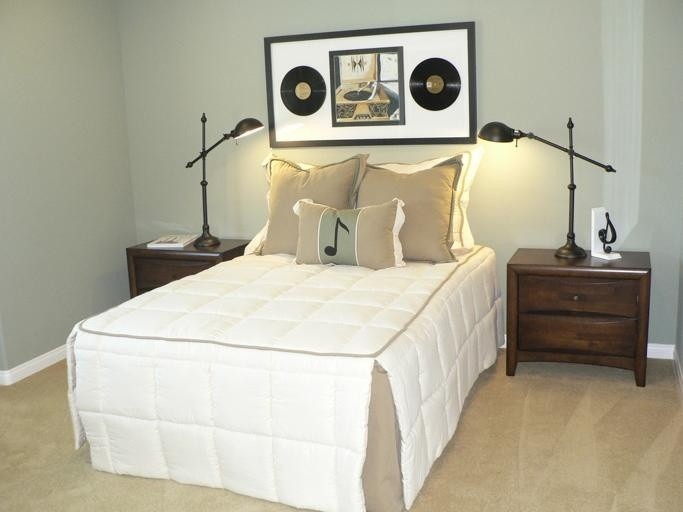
[263,20,478,148]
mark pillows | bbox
[258,153,369,258]
[242,153,318,254]
[366,152,483,255]
[293,197,407,269]
[354,153,466,265]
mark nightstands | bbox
[124,233,251,299]
[504,244,653,388]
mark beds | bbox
[58,151,513,512]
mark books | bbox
[147,233,201,248]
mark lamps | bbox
[479,113,619,259]
[184,106,263,248]
